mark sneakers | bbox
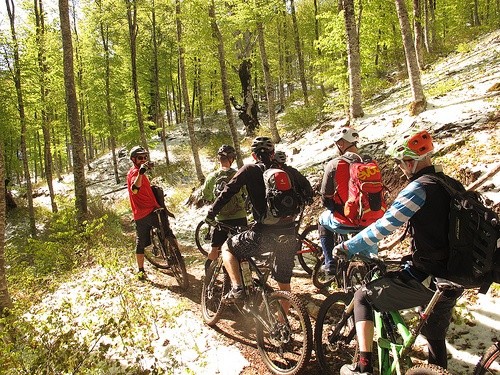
[340,362,373,375]
[270,320,292,340]
[221,288,245,304]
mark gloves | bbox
[205,215,216,226]
[332,240,347,262]
[139,161,149,174]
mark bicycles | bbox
[201,216,313,375]
[143,208,189,290]
[314,248,465,375]
[195,193,387,292]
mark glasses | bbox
[134,155,147,160]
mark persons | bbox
[274,151,314,204]
[127,145,177,280]
[205,136,315,341]
[202,144,252,292]
[318,126,378,277]
[332,129,466,375]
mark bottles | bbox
[240,258,254,287]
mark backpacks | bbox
[214,168,243,215]
[249,163,300,218]
[335,155,387,221]
[423,174,500,284]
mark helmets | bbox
[218,144,236,158]
[333,126,359,143]
[250,136,276,152]
[384,129,435,164]
[130,145,148,160]
[273,151,286,163]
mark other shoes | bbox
[320,265,336,275]
[201,275,211,285]
[138,272,147,280]
